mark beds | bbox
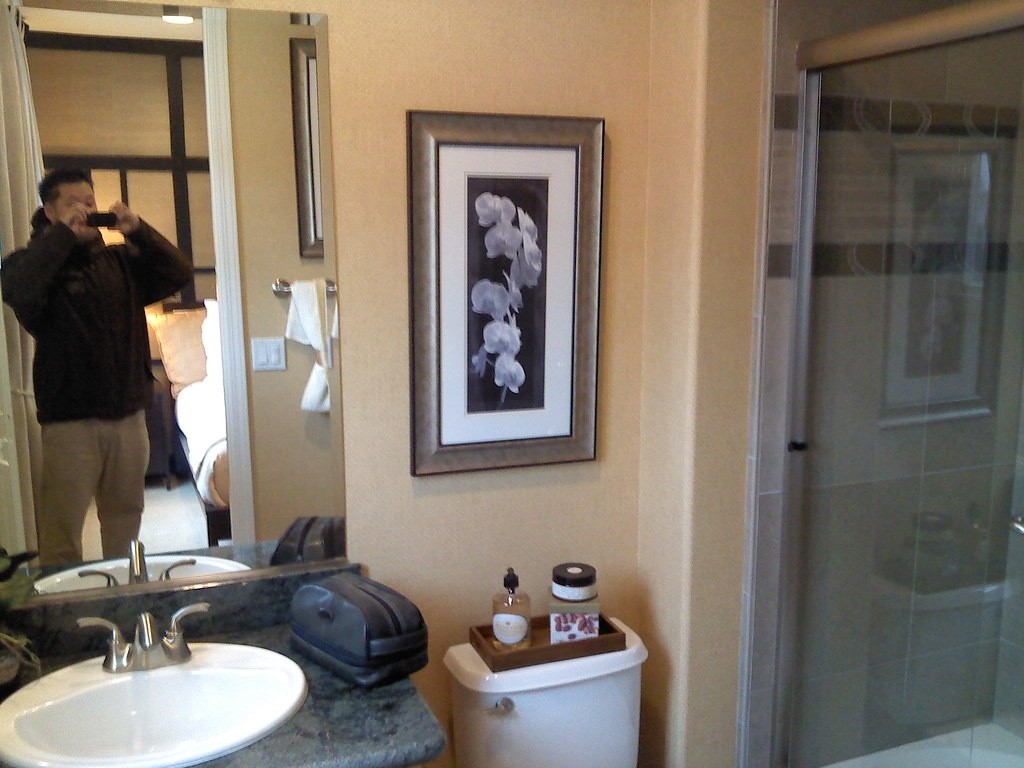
[152,297,231,546]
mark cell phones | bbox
[86,213,116,227]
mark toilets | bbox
[442,618,648,768]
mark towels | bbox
[284,279,340,414]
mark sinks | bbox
[0,666,308,767]
[35,555,252,592]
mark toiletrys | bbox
[492,567,532,651]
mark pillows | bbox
[147,311,208,398]
[200,299,225,380]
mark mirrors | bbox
[0,0,348,599]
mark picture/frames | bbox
[291,38,325,259]
[879,144,997,429]
[405,109,598,477]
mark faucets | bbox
[126,610,165,672]
[125,539,152,587]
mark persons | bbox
[0,168,195,566]
